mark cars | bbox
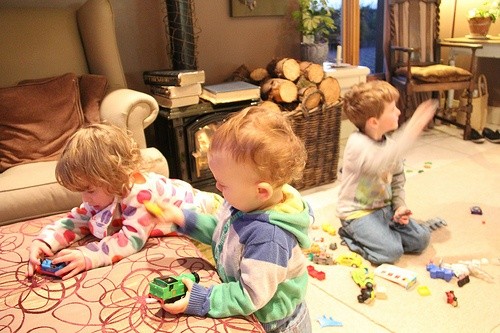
[471,206,483,215]
[444,290,458,306]
[334,252,364,268]
[358,283,376,303]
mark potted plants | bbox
[467,0,500,36]
[291,0,338,66]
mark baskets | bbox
[277,89,344,191]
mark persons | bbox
[156,107,314,333]
[334,80,438,264]
[28,123,224,277]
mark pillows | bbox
[0,73,86,174]
[17,74,110,125]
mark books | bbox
[201,80,260,105]
[150,86,202,108]
[143,69,205,86]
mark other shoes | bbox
[471,128,485,143]
[483,127,500,142]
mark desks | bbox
[153,98,264,199]
[323,61,370,138]
[442,35,500,110]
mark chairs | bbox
[387,0,483,141]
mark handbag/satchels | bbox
[456,74,488,130]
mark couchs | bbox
[0,0,170,229]
[0,212,266,333]
